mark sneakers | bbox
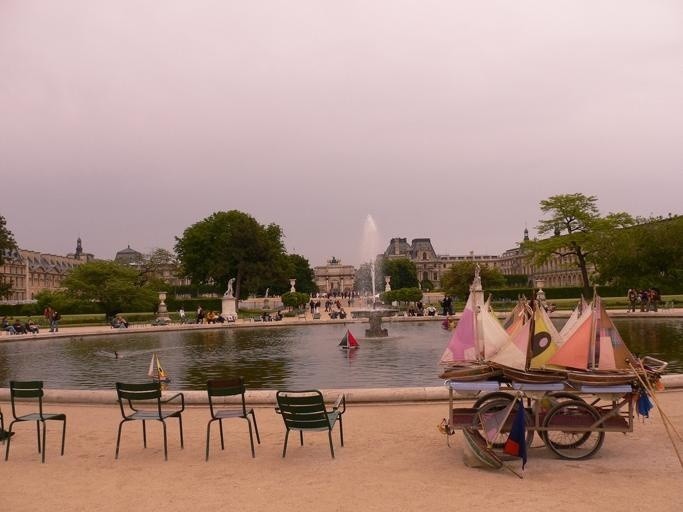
[11,328,59,335]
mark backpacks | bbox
[55,311,62,320]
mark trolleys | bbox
[441,352,667,459]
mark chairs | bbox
[274,388,345,459]
[7,380,67,463]
[202,377,260,464]
[115,382,185,461]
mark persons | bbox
[197,304,225,324]
[310,289,360,319]
[263,299,270,309]
[409,295,453,316]
[627,287,658,312]
[180,307,185,324]
[261,310,283,321]
[44,307,58,332]
[3,315,39,335]
[112,316,128,328]
